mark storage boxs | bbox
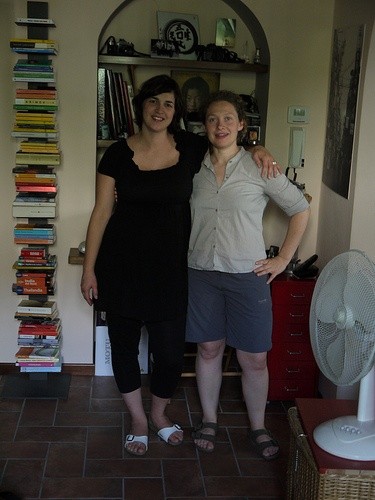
[94,311,149,376]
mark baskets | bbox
[283,407,375,500]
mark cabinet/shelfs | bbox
[0,1,72,399]
[267,274,319,402]
[96,56,268,149]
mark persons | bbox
[181,76,210,113]
[186,91,310,458]
[80,75,283,455]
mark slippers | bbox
[250,429,280,459]
[148,423,184,446]
[125,435,148,456]
[192,422,217,452]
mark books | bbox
[97,67,138,139]
[9,18,61,373]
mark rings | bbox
[272,160,277,165]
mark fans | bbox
[309,249,375,462]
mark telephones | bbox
[288,127,305,168]
